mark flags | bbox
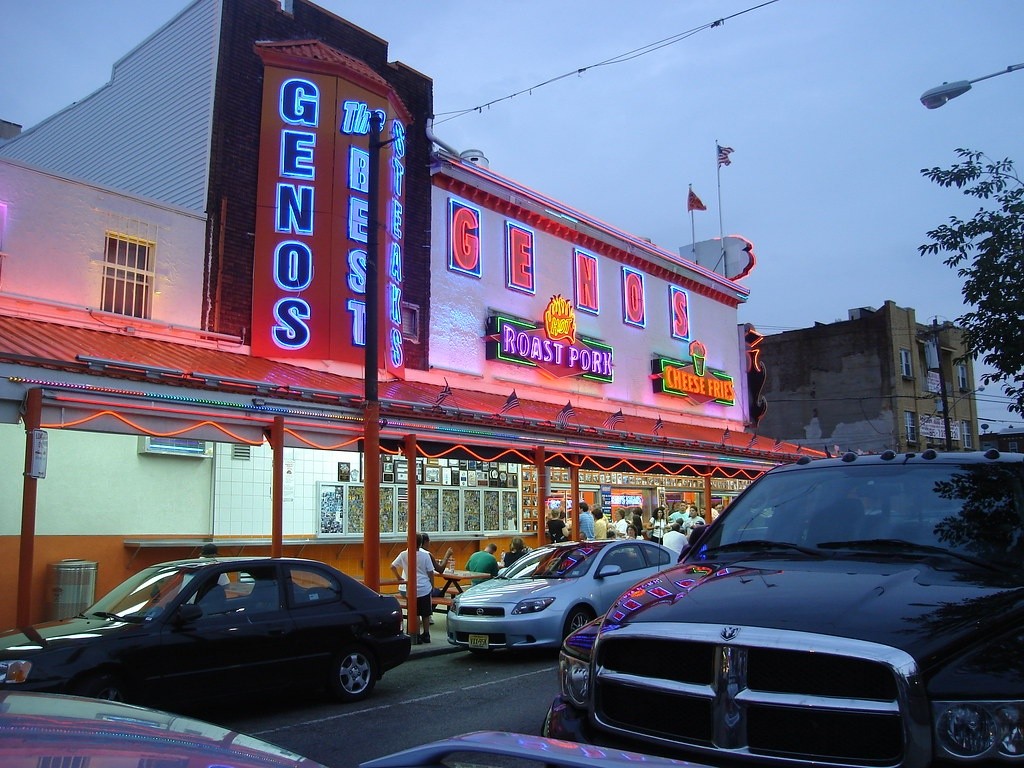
[717,145,734,168]
[687,189,706,211]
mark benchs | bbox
[440,588,466,594]
[395,593,452,605]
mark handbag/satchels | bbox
[647,519,656,538]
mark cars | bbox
[446,540,678,658]
[0,556,414,718]
[0,688,705,768]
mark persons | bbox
[390,533,434,644]
[504,536,526,568]
[660,501,724,542]
[398,533,453,624]
[591,509,606,539]
[663,524,689,554]
[606,506,647,540]
[646,508,670,544]
[179,543,230,604]
[592,503,609,530]
[547,509,569,543]
[465,543,499,585]
[579,502,595,540]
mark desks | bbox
[432,570,491,612]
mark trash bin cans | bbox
[44,557,99,624]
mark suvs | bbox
[543,449,1024,768]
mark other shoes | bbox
[429,616,434,624]
[417,634,422,645]
[422,632,430,644]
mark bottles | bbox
[450,559,455,574]
[501,549,505,564]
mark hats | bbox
[201,544,218,557]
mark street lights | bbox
[944,384,986,450]
